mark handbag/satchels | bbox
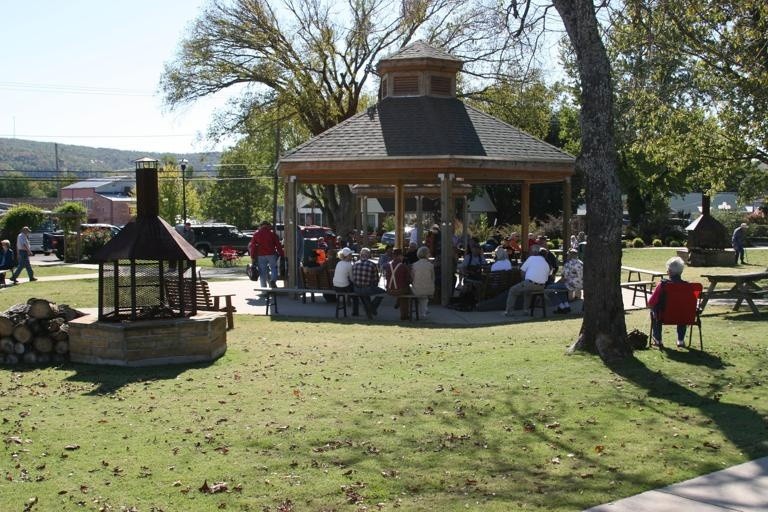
[246,263,260,281]
[627,328,649,350]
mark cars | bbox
[241,222,419,249]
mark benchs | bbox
[298,265,336,303]
[164,277,237,331]
[696,268,767,320]
[482,269,521,293]
[618,279,657,307]
[250,284,346,320]
[528,285,587,316]
[621,264,670,294]
[336,291,434,322]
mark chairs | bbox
[646,280,709,352]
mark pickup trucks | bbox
[43,224,122,263]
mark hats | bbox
[22,226,32,232]
[343,247,354,257]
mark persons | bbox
[648,257,697,347]
[732,223,750,264]
[250,221,284,296]
[184,223,195,267]
[10,226,37,282]
[0,239,14,287]
[295,224,583,320]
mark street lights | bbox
[178,158,189,223]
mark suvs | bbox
[175,223,255,257]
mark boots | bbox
[553,306,571,313]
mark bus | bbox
[0,208,54,256]
